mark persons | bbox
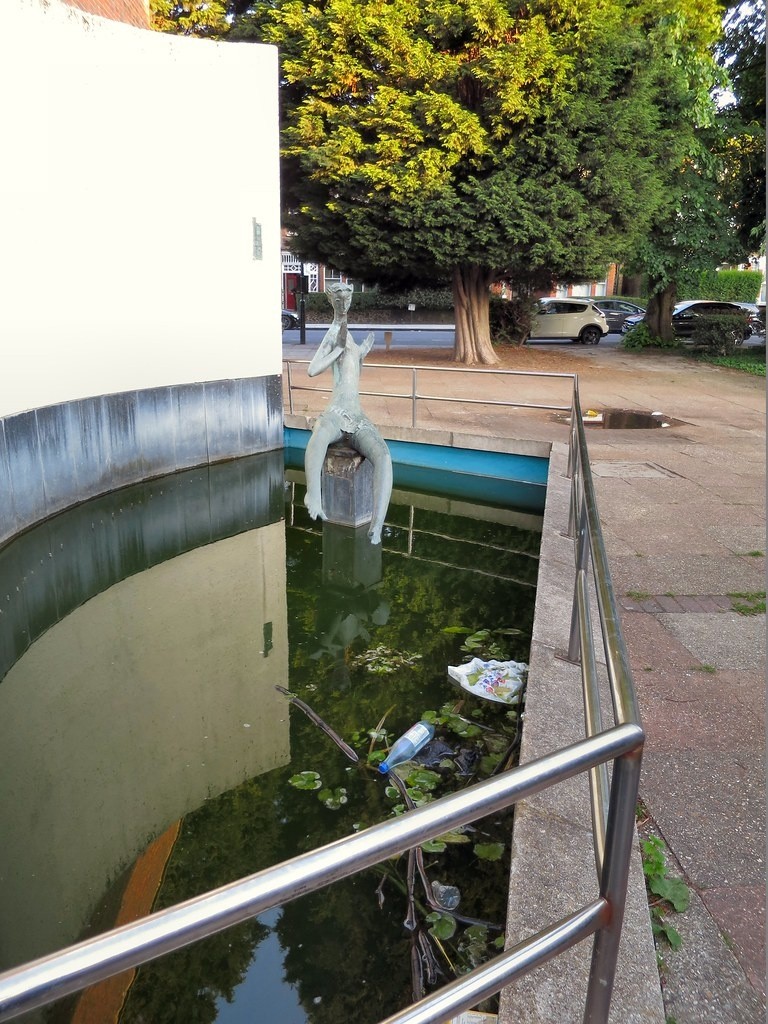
[304,282,393,545]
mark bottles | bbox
[379,720,435,773]
[432,884,460,910]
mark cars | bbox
[282,309,300,330]
[595,299,646,331]
[620,299,753,345]
[735,302,760,334]
[527,298,609,344]
